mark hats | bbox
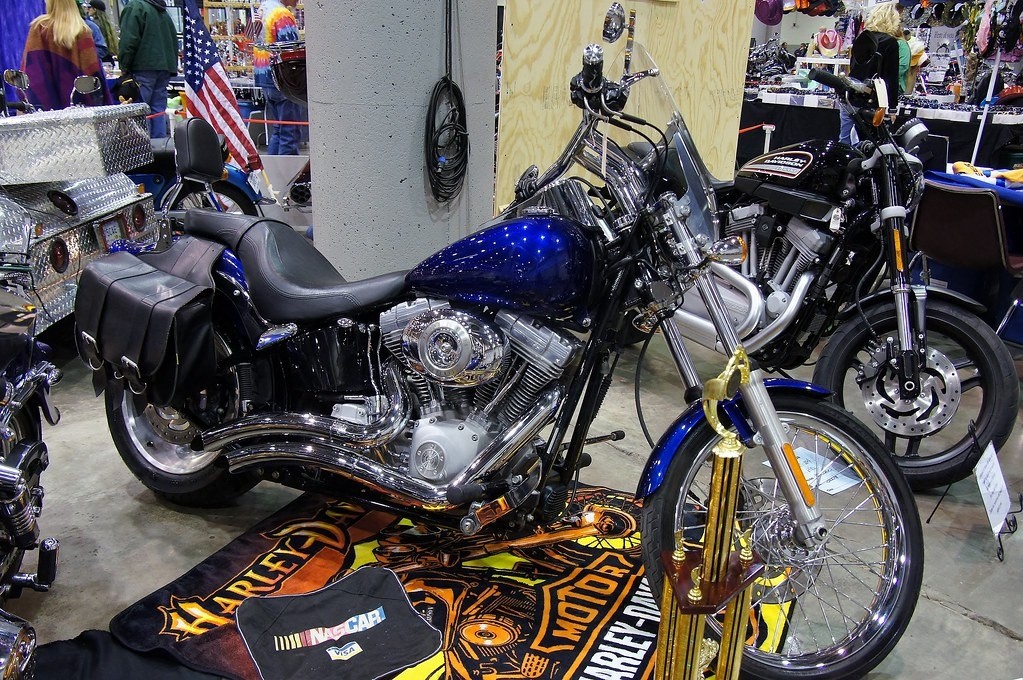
[994,85,1023,105]
[754,0,783,26]
[896,0,969,28]
[85,0,106,11]
[817,29,842,57]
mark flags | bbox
[245,7,262,40]
[183,0,262,175]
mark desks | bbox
[734,92,1023,171]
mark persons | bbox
[76,0,119,67]
[15,0,112,117]
[779,27,842,73]
[838,2,923,145]
[236,18,244,33]
[253,0,301,156]
[118,0,179,139]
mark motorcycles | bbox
[76,0,926,680]
[625,63,1023,492]
[0,248,63,680]
[0,68,279,369]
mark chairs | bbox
[908,178,1022,338]
